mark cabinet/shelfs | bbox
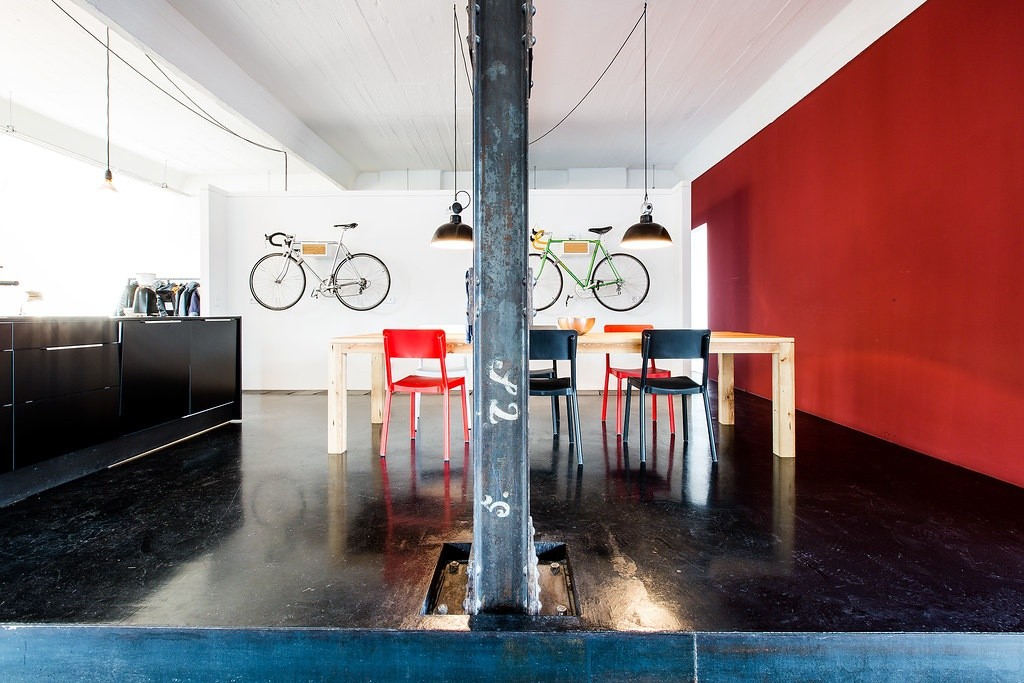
[0,317,14,476]
[14,315,121,471]
[121,315,242,438]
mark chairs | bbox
[601,325,675,435]
[623,329,718,463]
[530,328,584,465]
[379,329,469,462]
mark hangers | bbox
[130,277,193,294]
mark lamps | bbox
[431,2,472,243]
[101,24,116,193]
[621,1,672,243]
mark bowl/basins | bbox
[558,317,596,337]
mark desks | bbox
[328,331,795,458]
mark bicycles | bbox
[248,222,392,312]
[527,226,651,312]
